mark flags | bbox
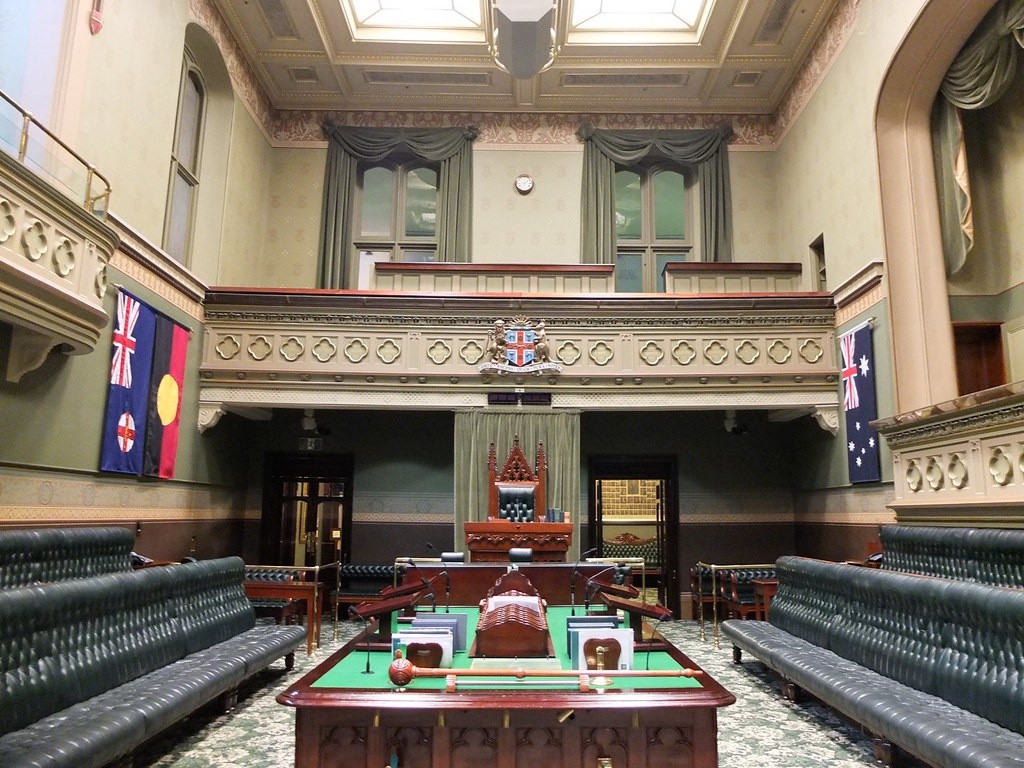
[141,310,190,480]
[100,287,157,475]
[837,319,882,483]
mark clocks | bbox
[515,174,534,194]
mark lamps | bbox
[480,0,575,79]
[724,410,737,433]
[302,409,315,430]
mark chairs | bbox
[441,552,464,562]
[509,547,533,561]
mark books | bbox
[390,613,468,668]
[565,616,635,670]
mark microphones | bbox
[514,499,519,522]
[571,548,625,616]
[646,615,669,670]
[349,605,370,673]
[410,542,450,613]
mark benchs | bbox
[722,556,1024,768]
[330,563,407,623]
[600,532,659,563]
[0,527,134,590]
[879,525,1024,590]
[691,559,776,624]
[243,567,305,626]
[0,556,307,768]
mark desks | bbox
[753,580,778,622]
[624,563,660,587]
[243,580,323,657]
[397,562,615,604]
[276,604,735,768]
